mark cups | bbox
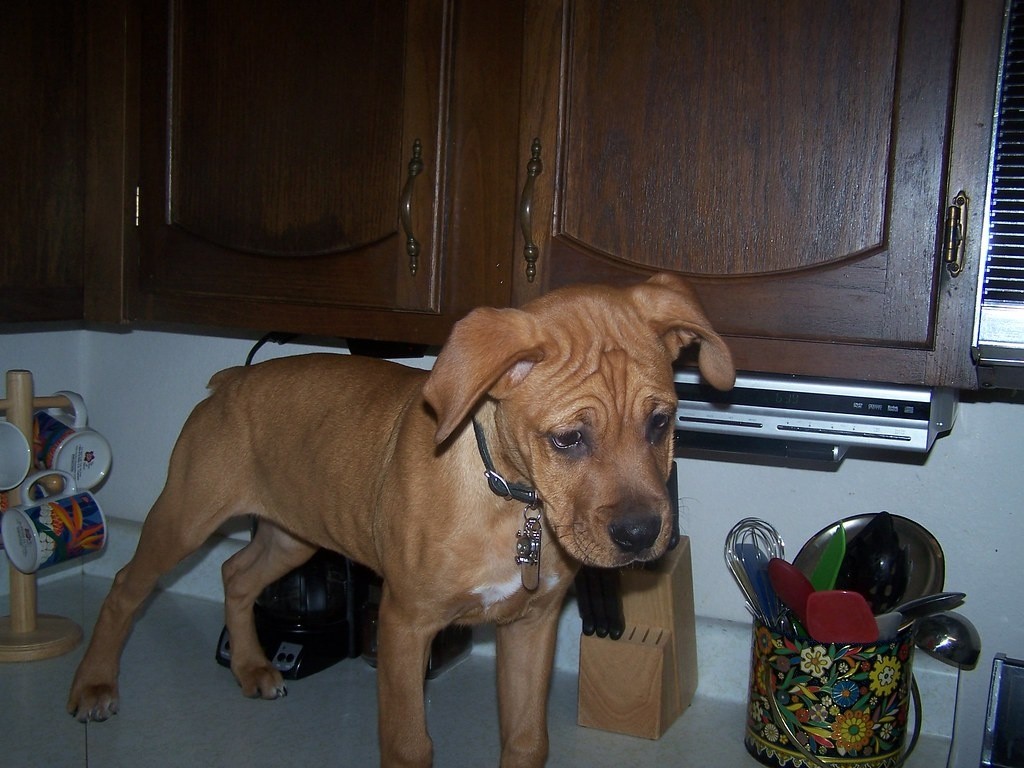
[32,390,112,490]
[0,420,31,491]
[0,467,108,577]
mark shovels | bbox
[806,592,879,644]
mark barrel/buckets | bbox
[745,615,922,768]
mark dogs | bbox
[66,272,736,768]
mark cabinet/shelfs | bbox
[1,1,1009,392]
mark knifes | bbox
[810,523,845,592]
[574,567,627,641]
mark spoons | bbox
[889,592,967,618]
